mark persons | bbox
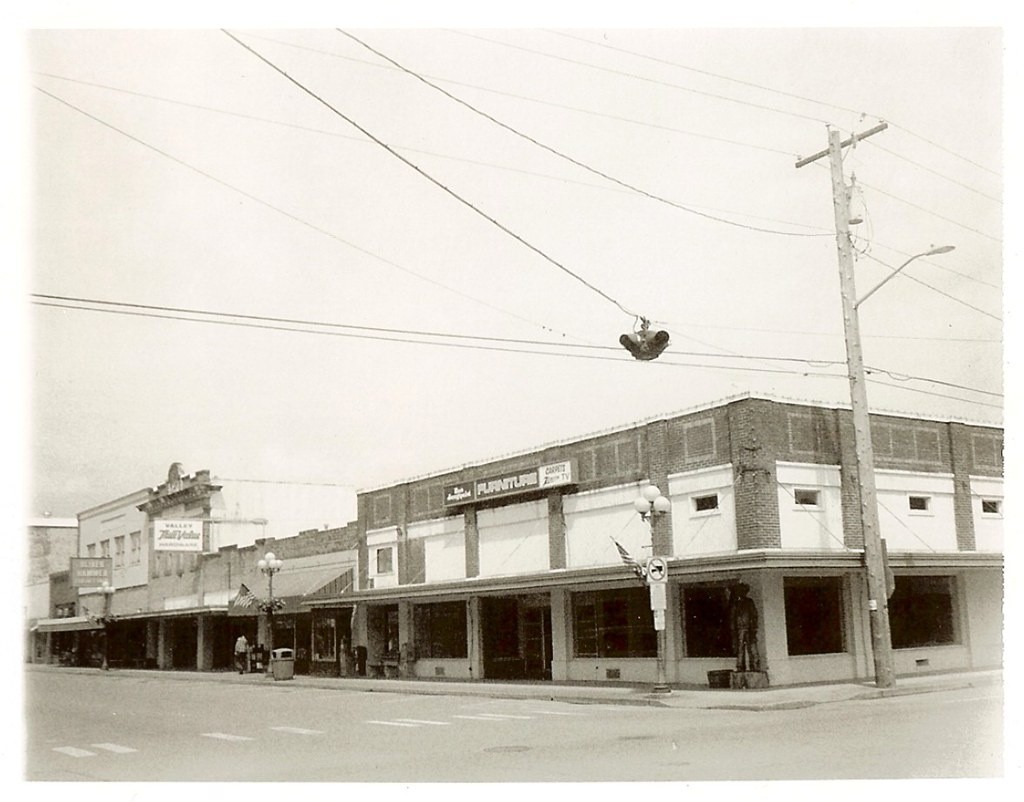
[235,632,248,674]
[730,583,759,672]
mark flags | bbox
[614,541,643,580]
[234,584,256,608]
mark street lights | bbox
[95,580,116,670]
[257,551,283,673]
[837,245,958,685]
[634,485,672,694]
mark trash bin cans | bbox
[271,648,294,681]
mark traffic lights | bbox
[619,329,643,361]
[643,329,670,361]
